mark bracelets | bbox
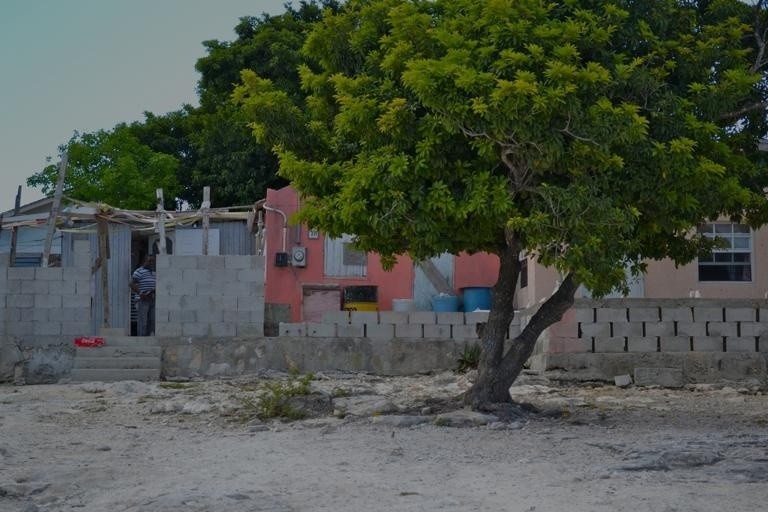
[138,291,142,296]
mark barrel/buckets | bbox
[342,285,379,310]
[463,289,493,313]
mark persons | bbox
[91,257,103,275]
[127,254,155,336]
